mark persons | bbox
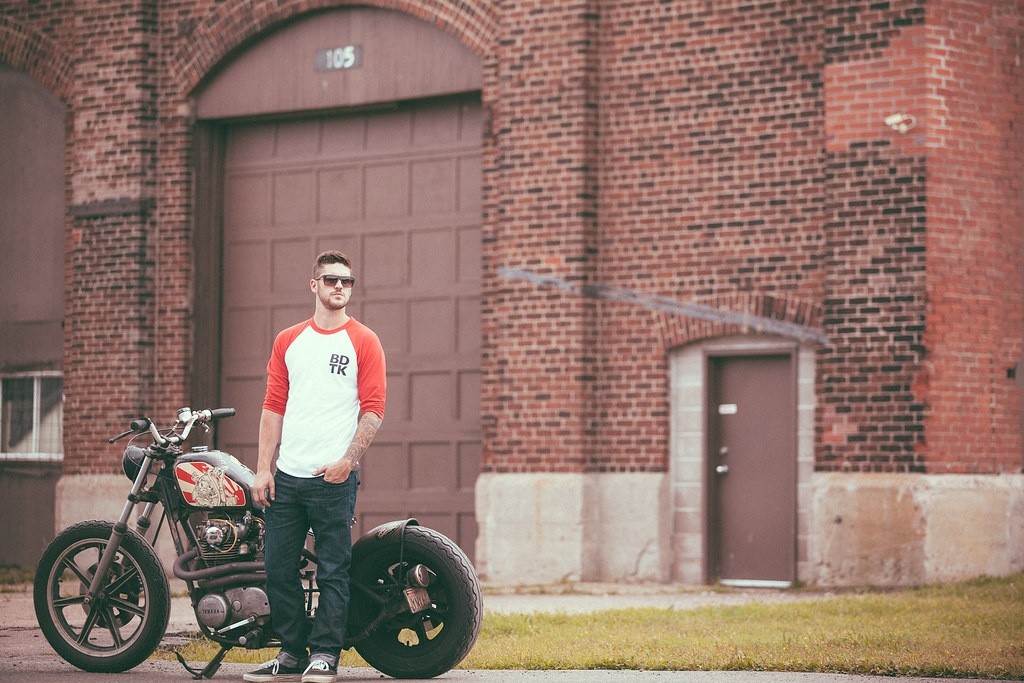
[241,249,387,682]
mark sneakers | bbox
[243,658,338,683]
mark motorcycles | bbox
[33,404,484,679]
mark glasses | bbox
[313,275,355,288]
